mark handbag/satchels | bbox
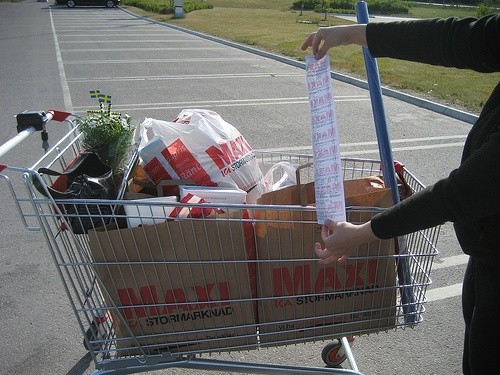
[138,108,262,203]
[256,162,395,347]
[36,151,121,236]
[87,179,259,356]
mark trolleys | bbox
[0,104,445,375]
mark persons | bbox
[301,14,500,375]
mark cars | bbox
[55,0,121,9]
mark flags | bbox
[89,89,100,99]
[98,94,112,104]
